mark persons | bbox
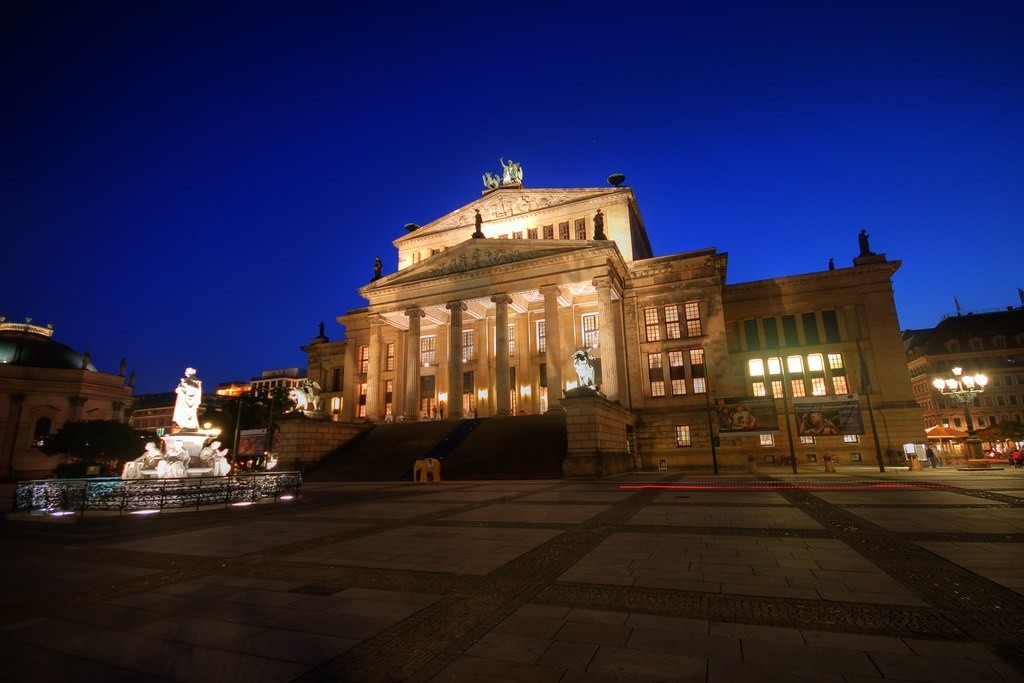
[433,400,445,421]
[926,446,937,468]
[124,441,232,479]
[1008,447,1024,469]
[173,367,202,431]
[829,258,835,270]
[858,230,872,256]
[473,407,479,419]
[499,157,522,184]
[799,413,841,436]
[593,209,605,235]
[722,405,757,431]
[318,320,324,336]
[129,371,135,387]
[82,349,91,370]
[475,209,483,233]
[119,358,127,377]
[373,256,383,279]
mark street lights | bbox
[932,365,992,471]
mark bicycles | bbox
[822,453,845,465]
[777,454,799,465]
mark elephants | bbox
[414,458,442,483]
